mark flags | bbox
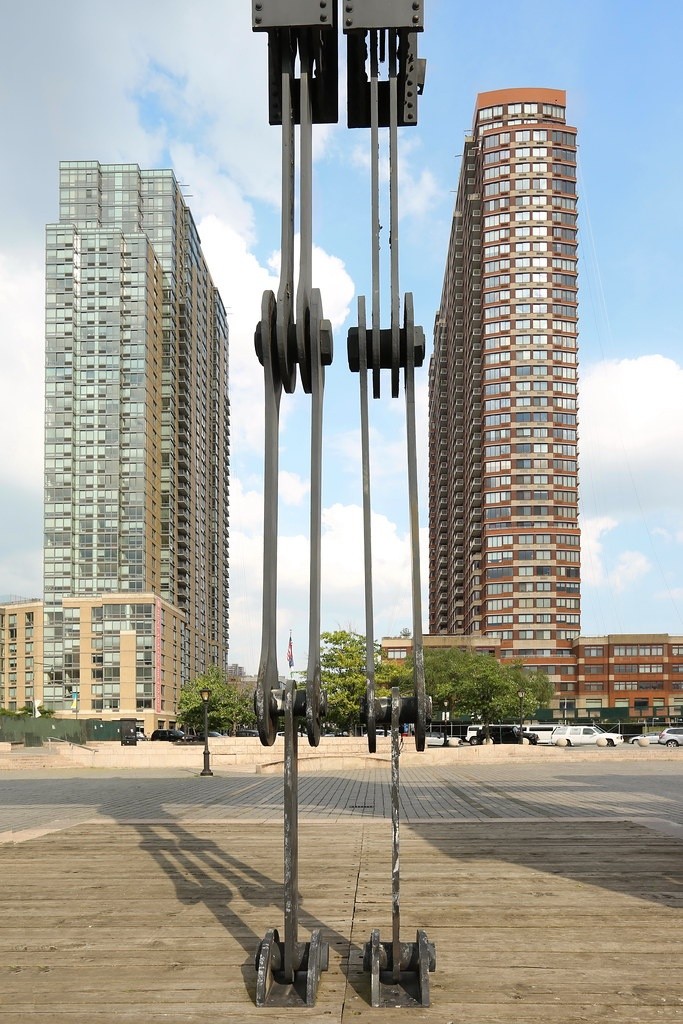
[286,638,294,667]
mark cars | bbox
[363,729,391,737]
[276,731,308,737]
[236,730,259,737]
[136,732,145,740]
[425,731,462,747]
[197,732,222,742]
[629,731,662,745]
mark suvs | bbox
[151,729,195,743]
[465,723,562,746]
[659,726,683,748]
[551,725,624,747]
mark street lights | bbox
[517,687,527,744]
[442,699,449,747]
[199,686,214,776]
